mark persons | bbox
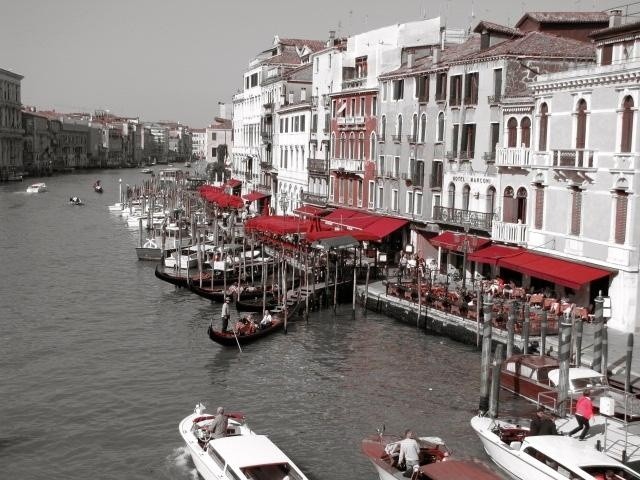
[604,470,615,480]
[221,281,272,336]
[567,389,593,442]
[399,254,407,275]
[526,409,544,456]
[394,428,422,471]
[207,406,229,439]
[484,274,505,298]
[535,413,559,464]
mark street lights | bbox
[461,216,471,288]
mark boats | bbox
[70,196,82,206]
[179,402,308,480]
[8,175,24,181]
[27,183,48,193]
[362,426,506,480]
[469,409,640,480]
[94,183,104,193]
[491,352,640,420]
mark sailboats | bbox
[108,160,303,349]
